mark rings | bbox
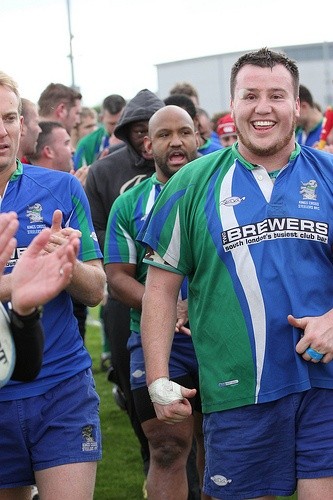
[59,267,74,279]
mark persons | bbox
[20,79,333,500]
[140,51,333,500]
[0,209,83,382]
[0,66,110,500]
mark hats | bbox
[216,114,238,138]
[318,108,333,148]
